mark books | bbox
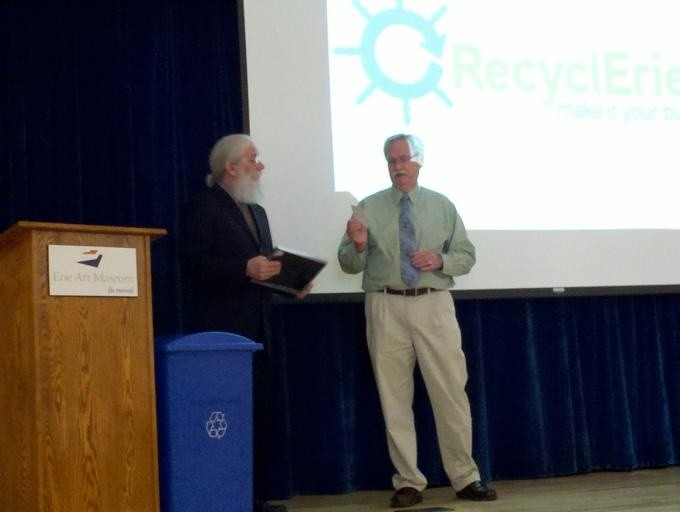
[251,246,328,299]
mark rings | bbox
[428,261,433,265]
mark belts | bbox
[376,288,434,296]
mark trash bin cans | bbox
[155,329,264,512]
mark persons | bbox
[179,133,314,512]
[338,133,497,505]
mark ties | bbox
[400,194,421,287]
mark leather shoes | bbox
[254,500,288,512]
[456,481,497,501]
[390,487,423,508]
